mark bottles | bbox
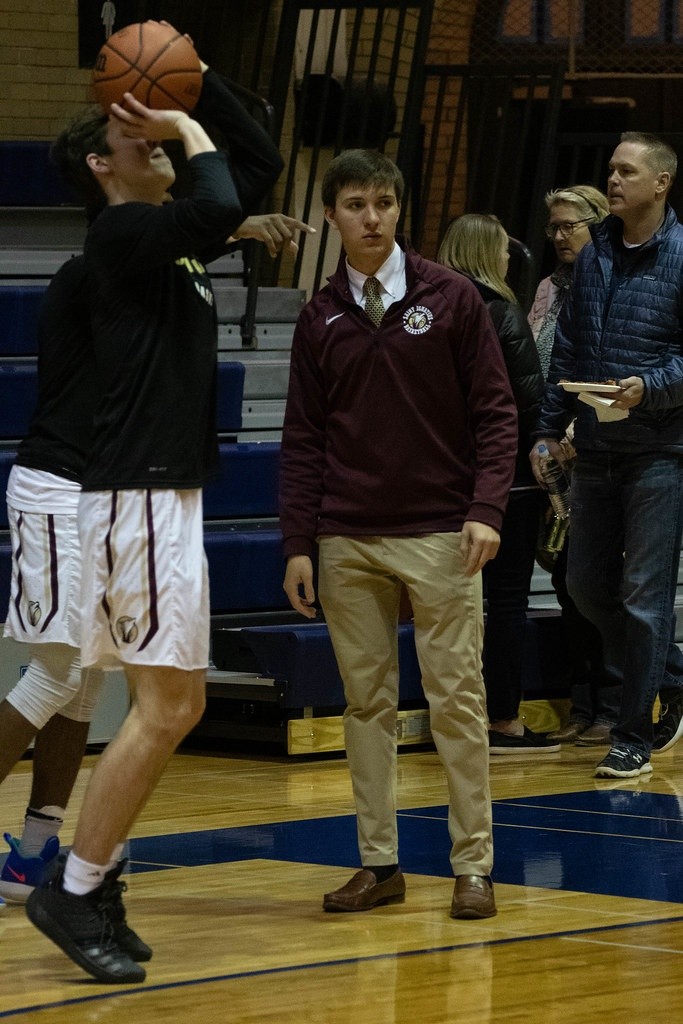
[537,443,573,517]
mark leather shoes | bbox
[322,868,407,911]
[548,718,613,747]
[452,873,496,918]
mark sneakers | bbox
[2,832,66,907]
[593,741,654,778]
[489,724,562,753]
[650,694,683,754]
[27,858,151,984]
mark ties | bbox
[364,279,384,326]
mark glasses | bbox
[545,217,595,236]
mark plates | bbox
[557,381,622,393]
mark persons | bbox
[434,213,562,753]
[527,184,622,746]
[278,148,519,920]
[0,187,316,903]
[534,130,681,778]
[24,20,283,984]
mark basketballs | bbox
[86,17,208,134]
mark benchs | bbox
[0,140,683,758]
